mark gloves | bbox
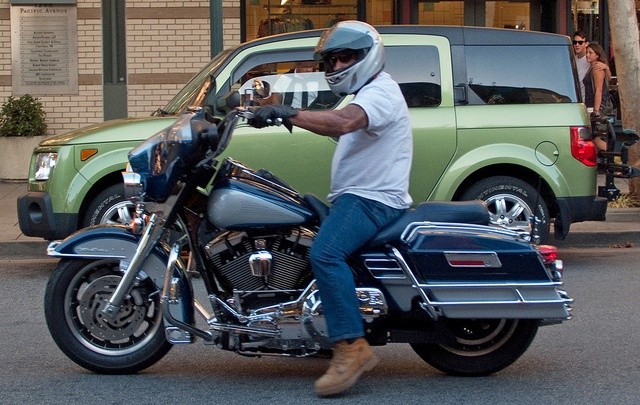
[247,104,298,134]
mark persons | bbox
[582,42,615,151]
[271,59,319,110]
[247,19,413,395]
[573,31,611,103]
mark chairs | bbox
[304,74,340,111]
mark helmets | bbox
[313,19,383,98]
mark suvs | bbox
[17,24,608,250]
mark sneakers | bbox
[313,338,379,396]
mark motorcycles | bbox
[41,73,575,379]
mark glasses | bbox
[322,49,354,65]
[572,40,586,45]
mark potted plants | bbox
[0,92,49,184]
